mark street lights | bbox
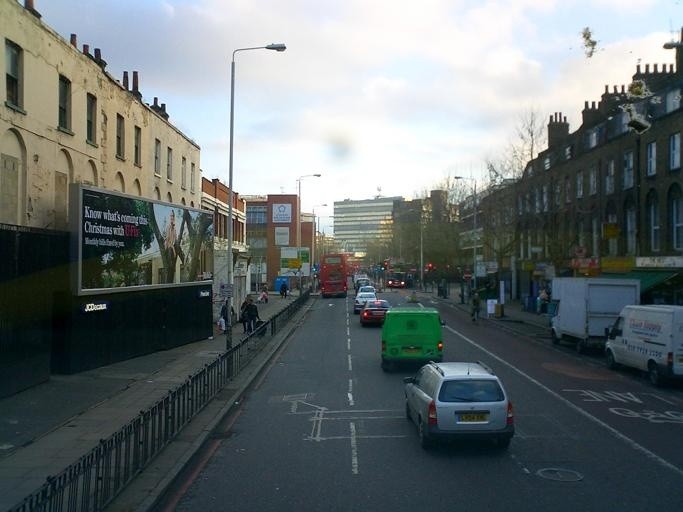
[295,172,322,296]
[225,38,289,383]
[453,175,480,290]
[408,209,427,290]
[312,201,334,277]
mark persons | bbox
[163,209,186,266]
[216,280,290,335]
[468,291,482,322]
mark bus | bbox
[346,262,360,276]
[318,251,348,299]
[385,270,405,289]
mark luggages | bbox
[255,320,266,336]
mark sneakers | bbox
[243,331,254,337]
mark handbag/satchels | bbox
[219,319,225,331]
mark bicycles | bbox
[469,307,481,324]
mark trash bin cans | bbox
[495,304,504,318]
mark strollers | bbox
[255,292,268,304]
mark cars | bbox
[350,271,390,328]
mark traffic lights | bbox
[428,262,433,270]
[381,267,385,271]
[384,261,388,266]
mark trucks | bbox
[548,275,644,356]
[601,302,683,389]
[379,304,446,372]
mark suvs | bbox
[402,359,514,452]
